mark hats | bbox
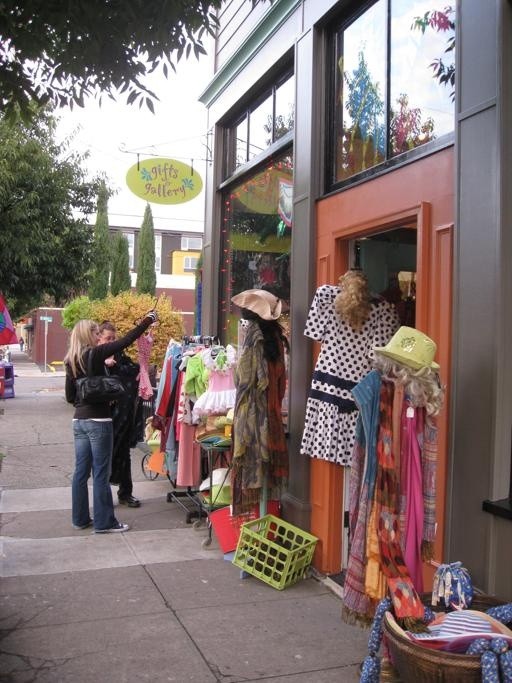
[374,325,440,369]
[229,289,283,320]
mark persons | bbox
[18,335,25,351]
[229,286,292,524]
[63,307,159,534]
[99,317,143,508]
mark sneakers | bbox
[119,495,140,507]
[96,523,129,533]
[72,518,94,529]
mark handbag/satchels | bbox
[75,375,126,401]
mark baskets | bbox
[233,513,319,591]
